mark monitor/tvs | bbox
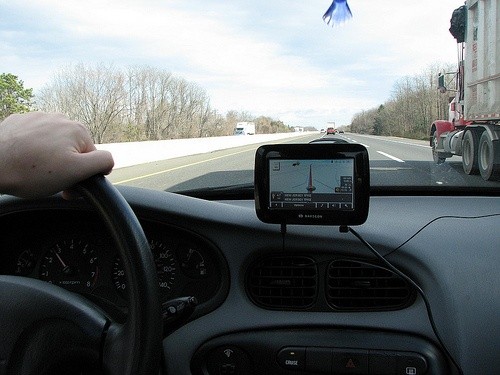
[254,144,370,226]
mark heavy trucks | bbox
[236,122,255,135]
[425,0,499,183]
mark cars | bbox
[321,129,325,133]
[339,129,344,133]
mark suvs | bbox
[327,128,335,135]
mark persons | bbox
[1,111,114,198]
